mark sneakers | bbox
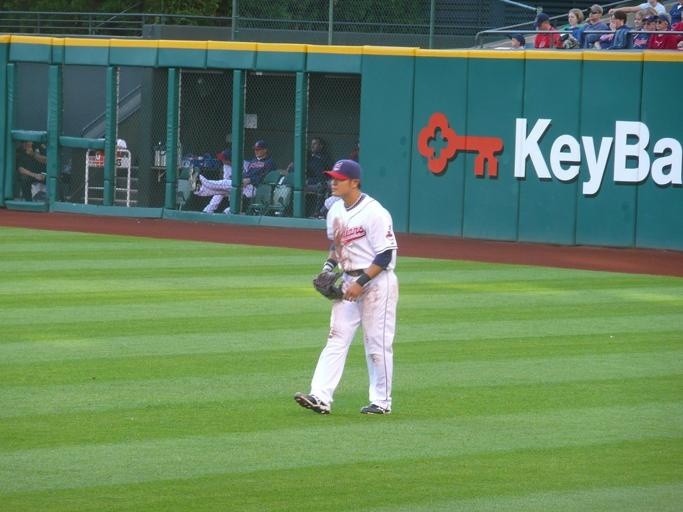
[294,391,331,415]
[189,164,202,192]
[359,403,391,415]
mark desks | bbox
[152,165,223,182]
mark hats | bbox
[587,5,604,14]
[322,159,362,180]
[506,34,525,44]
[252,140,268,150]
[642,13,668,22]
[532,13,549,27]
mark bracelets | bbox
[355,273,372,287]
[322,258,337,272]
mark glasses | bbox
[654,21,663,24]
[643,22,651,26]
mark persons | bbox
[292,159,400,415]
[188,137,360,220]
[504,0,682,50]
[16,141,73,204]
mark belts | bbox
[345,269,364,277]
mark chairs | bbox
[249,171,307,218]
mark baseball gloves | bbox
[312,270,344,300]
